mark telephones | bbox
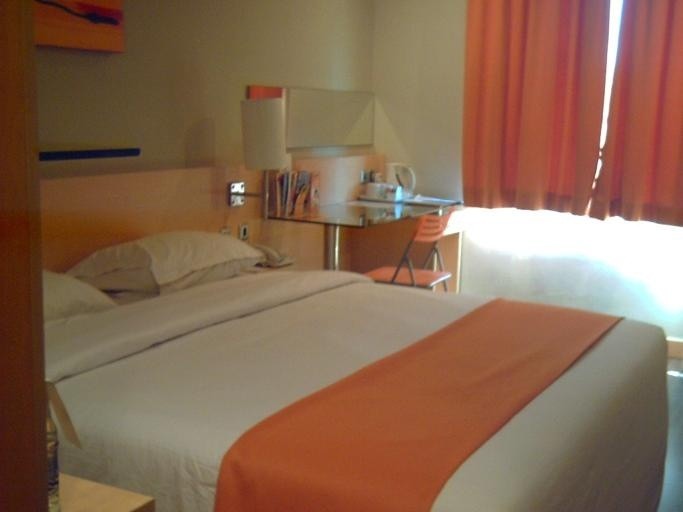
[255,244,293,268]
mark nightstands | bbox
[51,472,155,512]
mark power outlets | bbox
[360,170,370,183]
[220,229,233,235]
[239,224,250,240]
[369,170,377,182]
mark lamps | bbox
[239,97,289,221]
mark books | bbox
[403,193,462,210]
[270,170,321,218]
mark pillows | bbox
[65,229,269,295]
[40,268,120,326]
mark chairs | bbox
[360,206,456,291]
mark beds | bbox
[36,266,669,512]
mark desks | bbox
[268,196,462,272]
